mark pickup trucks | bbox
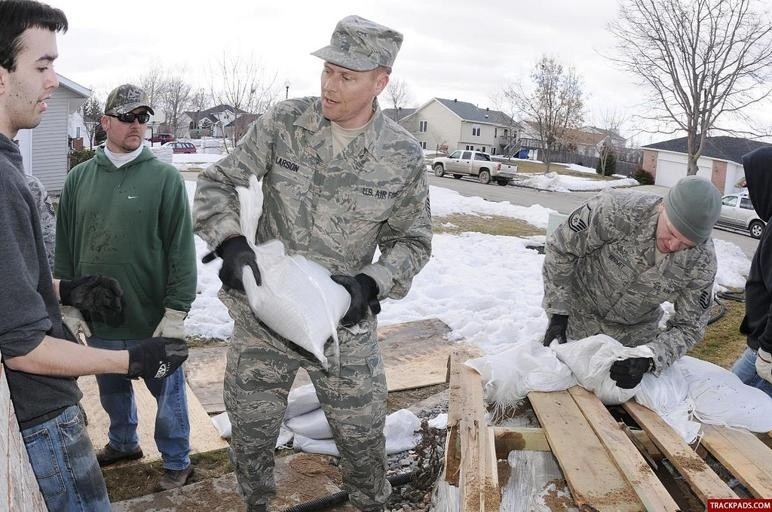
[431,150,519,186]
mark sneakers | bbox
[93,444,143,467]
[156,465,194,491]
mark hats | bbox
[663,175,722,244]
[104,84,154,115]
[309,14,403,72]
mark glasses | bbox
[109,112,150,125]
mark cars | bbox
[148,133,177,144]
[713,190,767,240]
[161,142,196,154]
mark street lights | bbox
[284,80,290,100]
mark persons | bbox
[541,173,722,390]
[52,83,196,491]
[193,13,433,512]
[731,146,772,399]
[0,0,189,510]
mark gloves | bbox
[331,273,380,328]
[755,353,772,383]
[58,274,125,345]
[543,315,569,346]
[202,236,261,290]
[123,308,188,381]
[609,358,655,389]
